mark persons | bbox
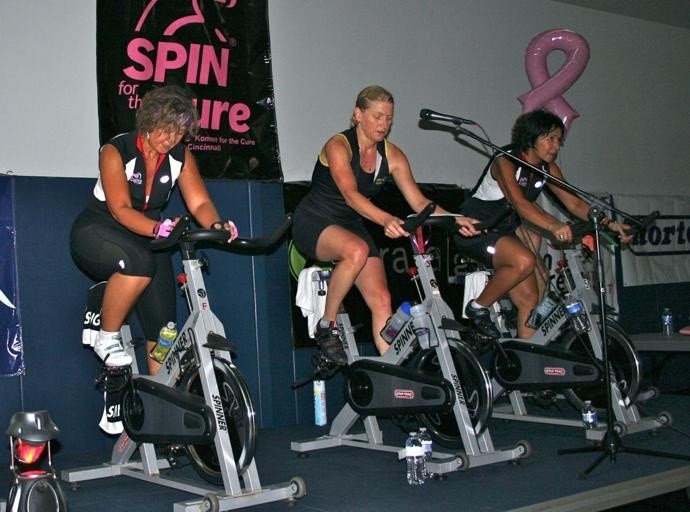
[291,86,486,365]
[455,109,633,343]
[68,87,238,375]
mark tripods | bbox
[455,126,690,481]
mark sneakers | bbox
[93,328,133,366]
[465,299,501,339]
[314,318,348,365]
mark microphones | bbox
[420,109,475,124]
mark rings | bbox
[560,234,565,239]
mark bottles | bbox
[418,427,434,480]
[404,432,424,487]
[661,308,674,336]
[564,292,592,334]
[582,400,599,446]
[151,321,178,362]
[525,290,561,331]
[380,301,414,345]
[312,365,329,427]
